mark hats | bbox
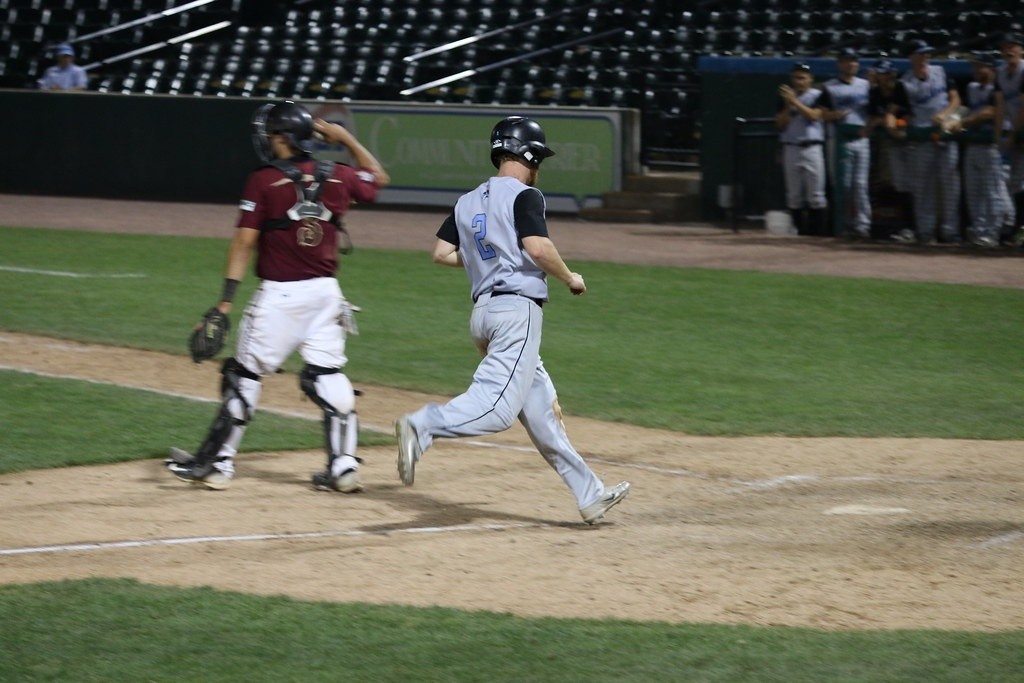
[971,54,995,66]
[1001,32,1024,45]
[793,62,812,74]
[905,40,934,54]
[873,61,897,76]
[57,43,74,58]
[838,48,857,59]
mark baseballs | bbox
[313,121,325,141]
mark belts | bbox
[475,291,544,308]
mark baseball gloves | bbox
[186,306,233,364]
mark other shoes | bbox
[843,225,1024,251]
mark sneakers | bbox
[165,459,230,490]
[396,419,421,485]
[579,482,630,525]
[312,474,358,493]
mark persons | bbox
[395,117,631,526]
[775,31,1024,248]
[163,103,389,493]
[39,44,88,92]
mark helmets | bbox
[491,117,555,167]
[264,101,314,154]
